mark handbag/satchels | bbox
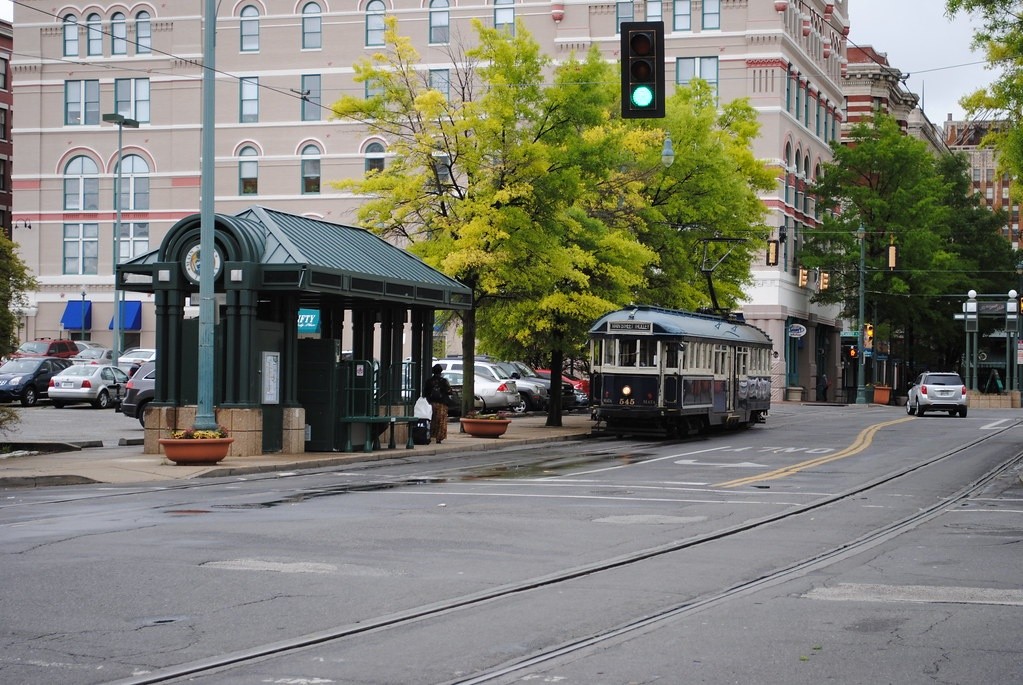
[443,391,459,405]
[414,396,433,420]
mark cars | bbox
[47,364,130,409]
[537,369,589,404]
[0,357,74,406]
[74,340,105,351]
[403,371,520,413]
[68,347,155,364]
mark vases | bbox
[460,418,511,438]
[159,438,234,465]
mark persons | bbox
[422,364,453,445]
[821,374,829,402]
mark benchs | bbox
[341,414,418,452]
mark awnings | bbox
[109,301,141,330]
[60,300,91,330]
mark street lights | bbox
[79,285,88,340]
[856,223,868,405]
[103,113,139,365]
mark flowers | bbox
[164,427,229,440]
[465,410,508,419]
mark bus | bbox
[588,306,772,440]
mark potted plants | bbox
[874,383,892,404]
[894,392,908,406]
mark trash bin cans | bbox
[410,418,431,445]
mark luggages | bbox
[408,418,431,445]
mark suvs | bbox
[115,361,156,428]
[483,360,576,412]
[432,359,549,414]
[0,338,79,368]
[906,372,968,419]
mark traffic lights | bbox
[850,349,859,359]
[620,23,667,117]
